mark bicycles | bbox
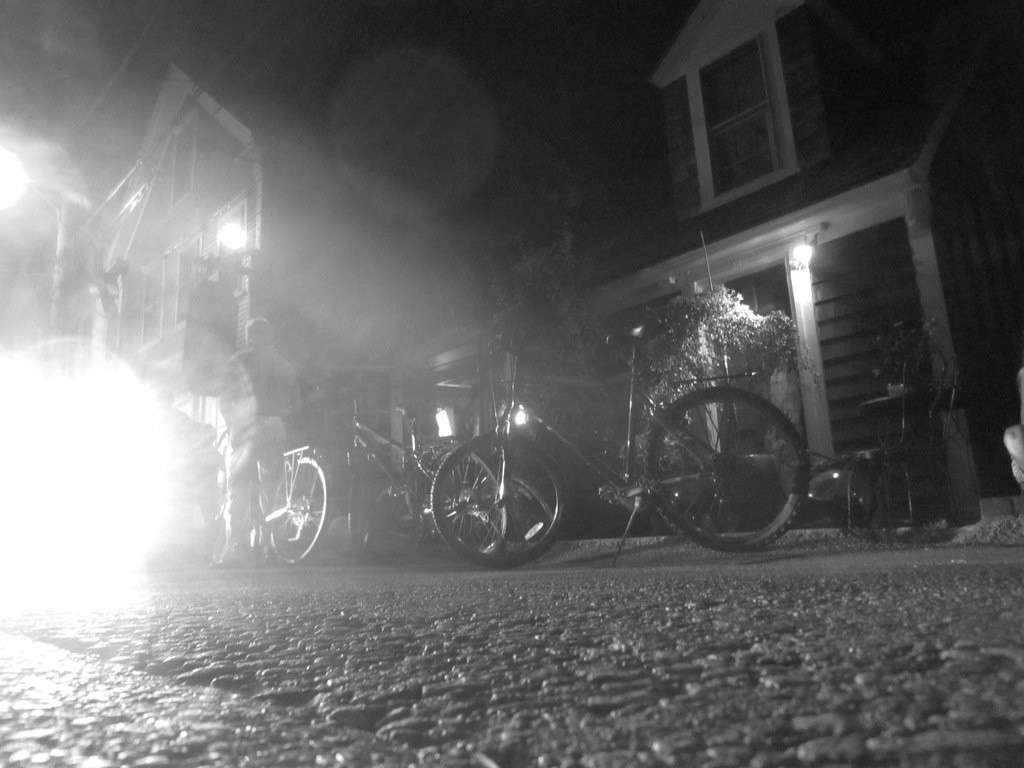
[209,317,812,569]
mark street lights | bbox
[1,148,69,337]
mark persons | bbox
[219,318,301,572]
